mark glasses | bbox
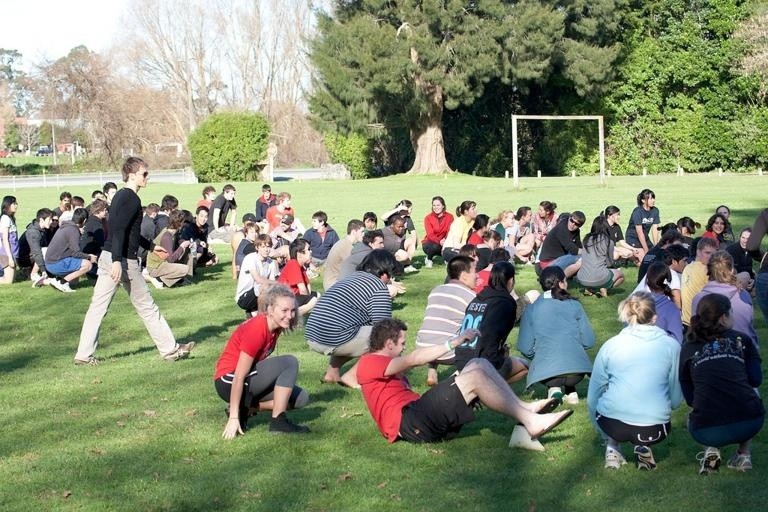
[569,217,581,228]
[302,246,312,251]
[12,201,19,206]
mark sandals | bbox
[583,290,595,297]
[595,289,607,300]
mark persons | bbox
[0,158,767,473]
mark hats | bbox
[242,213,259,224]
[281,214,295,223]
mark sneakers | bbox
[601,442,629,472]
[149,277,166,291]
[509,424,549,458]
[72,352,103,368]
[423,257,433,269]
[162,341,196,362]
[725,451,752,474]
[403,264,419,277]
[425,369,440,388]
[137,171,149,178]
[632,445,658,473]
[268,412,310,437]
[224,407,250,431]
[695,446,722,483]
[545,387,562,407]
[32,276,45,291]
[50,279,77,293]
[561,391,579,406]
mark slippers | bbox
[337,377,363,395]
[319,372,342,385]
[516,398,559,426]
[532,410,575,440]
